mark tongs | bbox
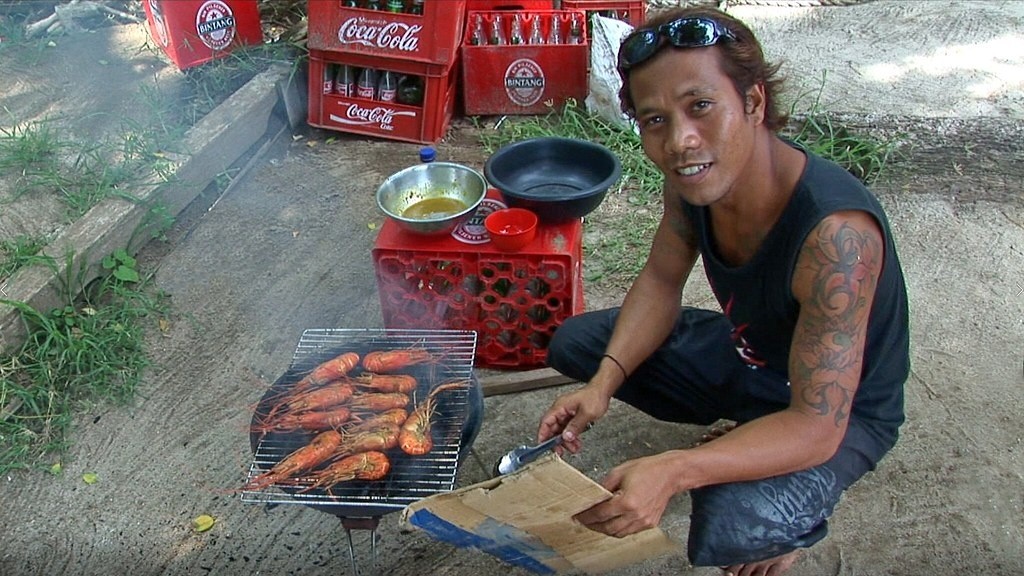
[494,420,594,479]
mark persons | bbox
[536,7,910,576]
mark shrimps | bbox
[362,348,431,373]
[400,379,474,455]
[220,431,341,493]
[349,391,410,412]
[293,451,390,492]
[333,407,408,459]
[251,352,359,430]
[351,371,418,393]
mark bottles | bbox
[608,10,618,20]
[471,13,579,46]
[321,0,424,107]
[419,147,436,163]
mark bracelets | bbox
[604,355,627,379]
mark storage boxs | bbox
[371,188,583,370]
[305,0,646,146]
[141,0,263,71]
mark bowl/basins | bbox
[485,208,538,252]
[376,161,488,236]
[483,136,622,224]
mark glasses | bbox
[613,15,749,83]
